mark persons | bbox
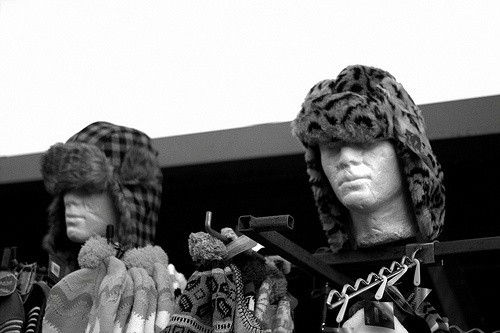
[41,122,163,266]
[298,62,447,253]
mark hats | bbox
[42,122,164,274]
[0,228,451,333]
[291,64,447,255]
